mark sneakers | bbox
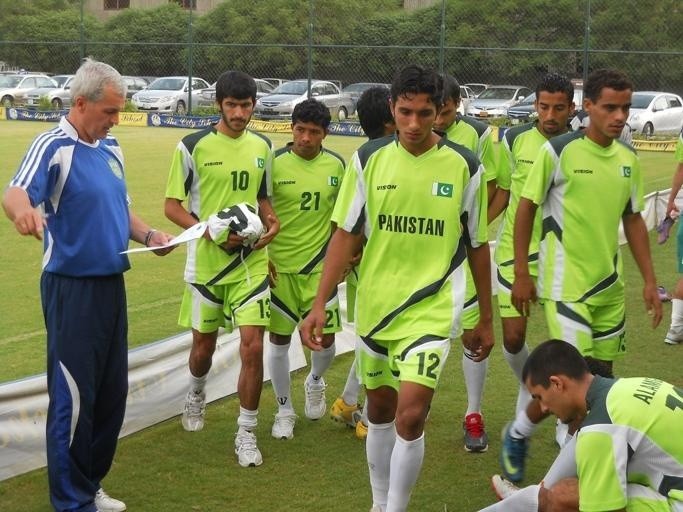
[234,427,264,468]
[271,411,299,442]
[461,412,490,454]
[181,390,206,433]
[328,396,363,428]
[498,420,531,483]
[355,421,368,439]
[304,375,327,421]
[491,474,522,500]
[657,286,672,302]
[656,210,674,245]
[93,487,126,512]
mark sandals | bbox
[663,328,683,346]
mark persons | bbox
[299,67,682,512]
[262,100,344,439]
[165,71,284,465]
[2,56,178,512]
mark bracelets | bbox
[144,228,156,247]
